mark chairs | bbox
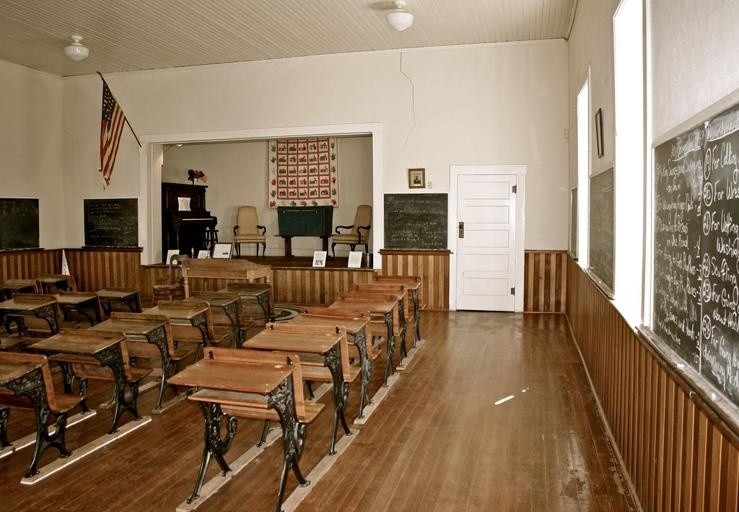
[186,291,255,348]
[330,205,371,261]
[51,290,104,330]
[152,255,187,305]
[288,306,381,419]
[0,351,88,478]
[329,293,403,388]
[243,323,361,457]
[350,282,414,357]
[233,206,267,256]
[0,335,30,352]
[94,288,143,313]
[372,276,427,349]
[167,347,325,512]
[25,328,153,435]
[140,299,233,399]
[0,278,42,300]
[91,311,194,416]
[1,293,63,336]
[219,284,281,329]
[34,274,77,292]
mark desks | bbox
[182,258,274,306]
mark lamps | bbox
[64,36,89,62]
[386,1,414,32]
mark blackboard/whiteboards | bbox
[588,165,615,288]
[650,89,738,407]
[84,198,138,248]
[571,187,577,260]
[384,193,448,250]
[0,198,40,250]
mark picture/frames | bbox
[213,242,233,259]
[409,168,425,188]
[348,252,362,269]
[198,250,210,259]
[166,250,179,264]
[312,251,327,267]
[595,108,604,158]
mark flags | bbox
[94,72,141,191]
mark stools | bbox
[204,229,219,252]
